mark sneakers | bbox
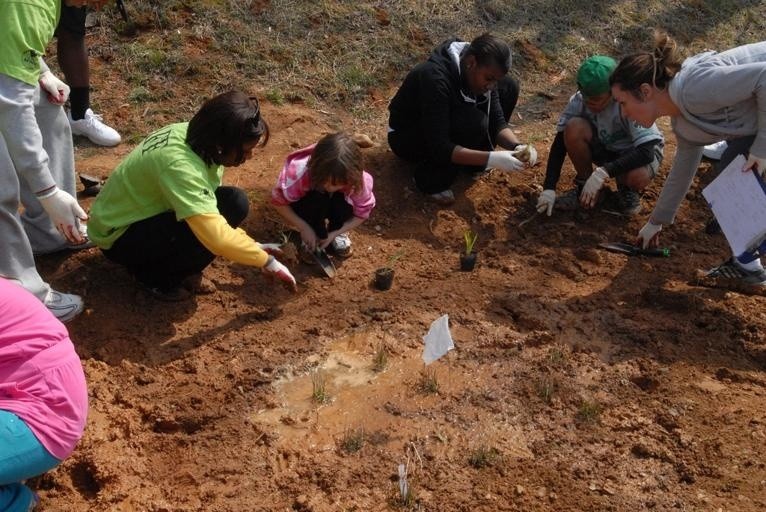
[66,223,97,249]
[300,240,319,265]
[327,230,353,258]
[553,169,642,214]
[132,273,217,302]
[428,189,455,206]
[67,108,121,147]
[703,140,729,160]
[46,288,85,325]
[692,257,766,289]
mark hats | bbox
[576,56,617,95]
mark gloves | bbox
[38,71,71,105]
[32,185,89,243]
[635,216,663,260]
[536,189,556,216]
[486,143,538,173]
[256,241,298,294]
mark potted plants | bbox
[459,228,480,272]
[374,247,405,291]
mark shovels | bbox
[305,244,337,279]
[598,241,669,257]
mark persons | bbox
[53,0,121,147]
[535,55,665,217]
[608,35,766,287]
[386,34,538,205]
[0,276,88,512]
[270,133,376,264]
[86,90,299,301]
[0,0,89,323]
